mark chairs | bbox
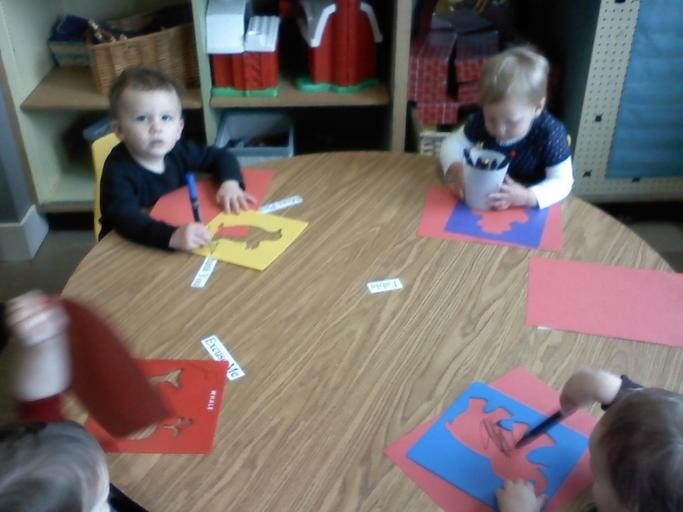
[88,132,131,241]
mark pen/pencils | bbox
[517,405,577,450]
[464,147,517,169]
[185,170,202,225]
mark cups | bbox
[461,149,510,209]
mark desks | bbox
[52,151,681,512]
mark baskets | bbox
[85,5,199,95]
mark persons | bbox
[96,63,259,253]
[432,44,574,215]
[495,367,683,511]
[0,287,118,511]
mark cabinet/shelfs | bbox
[1,1,605,216]
